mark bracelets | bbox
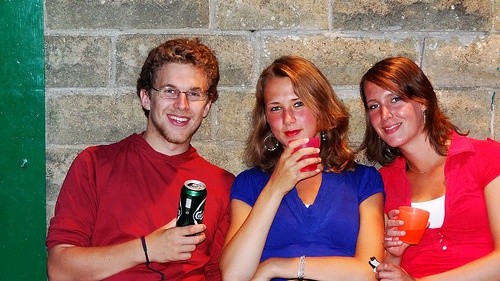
[297,255,305,279]
[141,235,164,281]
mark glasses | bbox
[151,85,209,101]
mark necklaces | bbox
[407,160,427,176]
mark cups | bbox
[289,138,321,172]
[397,206,430,245]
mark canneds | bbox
[176,180,207,236]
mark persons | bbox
[45,38,235,281]
[355,56,500,280]
[219,55,386,281]
